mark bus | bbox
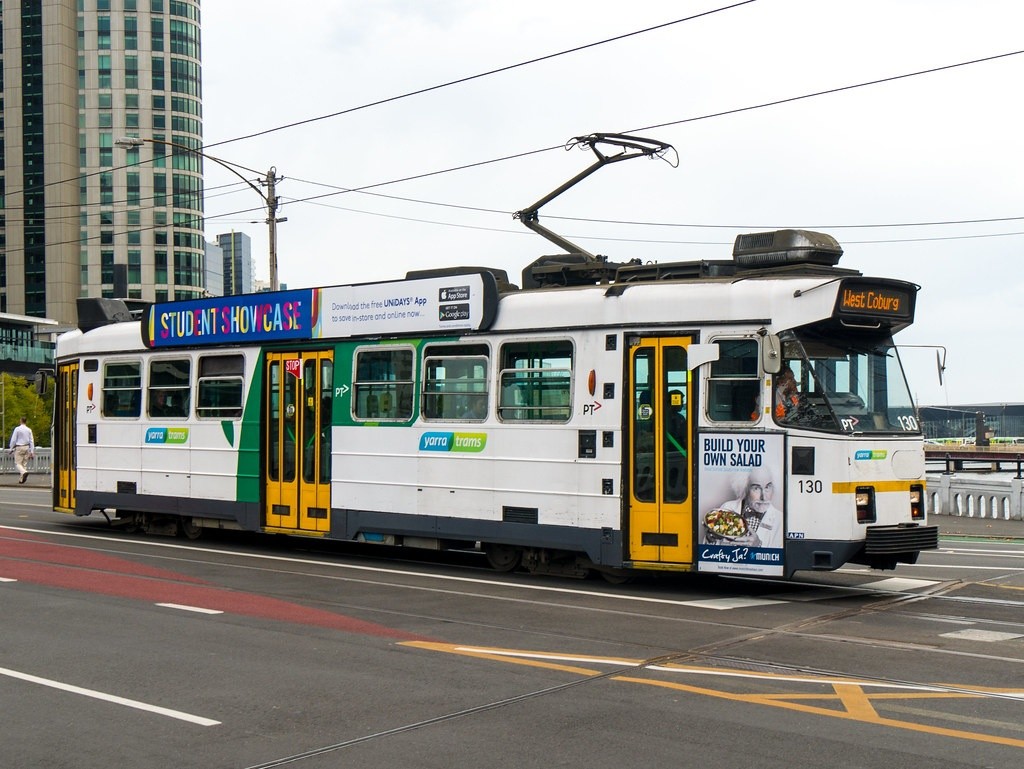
[924,437,1024,446]
[33,230,947,588]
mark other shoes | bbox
[23,472,29,483]
[19,480,23,484]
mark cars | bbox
[960,441,975,450]
[925,441,944,448]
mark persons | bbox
[751,370,800,421]
[668,389,686,442]
[461,395,485,419]
[9,417,34,484]
[702,464,783,548]
[150,391,171,417]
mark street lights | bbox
[115,138,277,290]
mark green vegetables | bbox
[706,509,743,536]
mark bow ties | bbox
[744,511,764,520]
